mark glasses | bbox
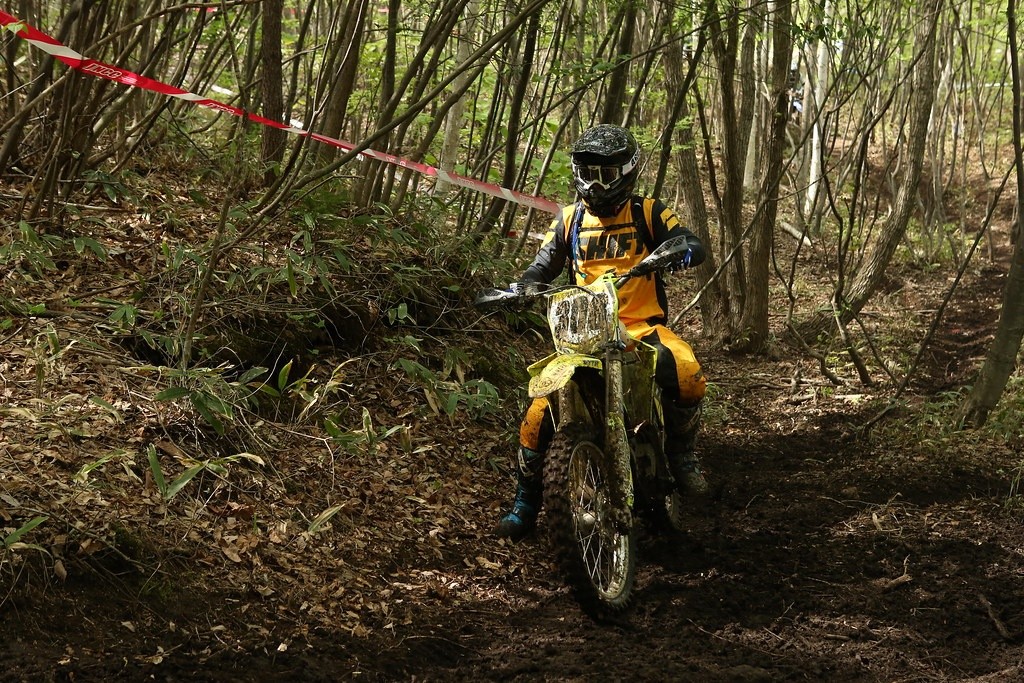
[572,165,621,186]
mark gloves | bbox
[498,283,535,312]
[664,243,693,275]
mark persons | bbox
[492,124,708,540]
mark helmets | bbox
[570,123,641,217]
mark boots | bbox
[495,445,544,539]
[661,391,709,496]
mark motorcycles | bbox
[477,236,702,624]
